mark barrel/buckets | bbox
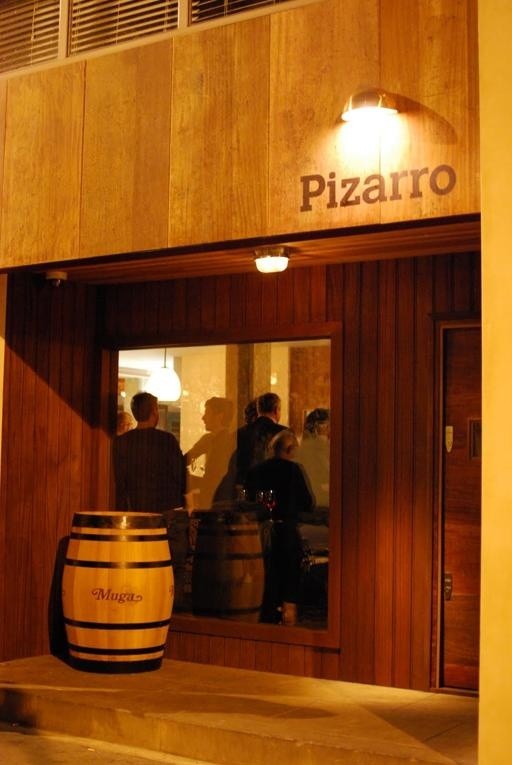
[60,508,174,663]
[192,506,269,622]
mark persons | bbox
[241,397,259,423]
[181,395,237,512]
[115,411,133,436]
[242,428,316,627]
[237,391,288,450]
[289,405,330,508]
[113,389,194,610]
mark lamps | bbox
[250,245,290,274]
[340,88,399,121]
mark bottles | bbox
[236,489,280,515]
